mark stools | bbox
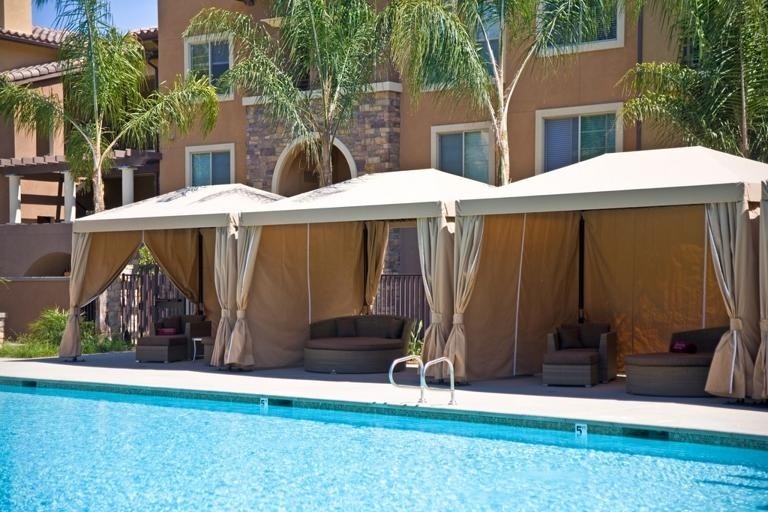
[542,351,600,389]
[135,335,187,364]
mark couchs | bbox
[622,326,731,398]
[304,315,412,374]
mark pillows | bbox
[558,327,599,349]
[335,318,404,339]
[157,315,182,335]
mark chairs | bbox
[546,321,617,383]
[148,314,211,361]
